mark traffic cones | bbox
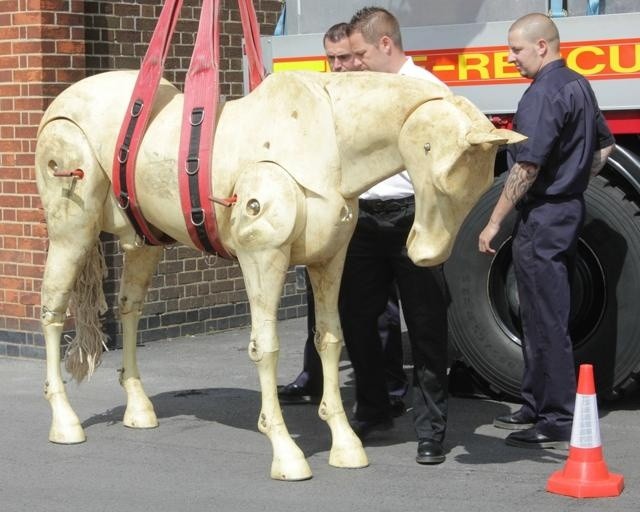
[546,364,625,500]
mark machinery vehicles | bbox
[241,0,639,410]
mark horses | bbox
[33,67,530,482]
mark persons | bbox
[335,5,451,466]
[272,21,411,411]
[477,10,618,449]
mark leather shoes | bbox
[415,438,447,464]
[492,409,573,450]
[275,384,323,404]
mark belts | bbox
[357,195,414,213]
